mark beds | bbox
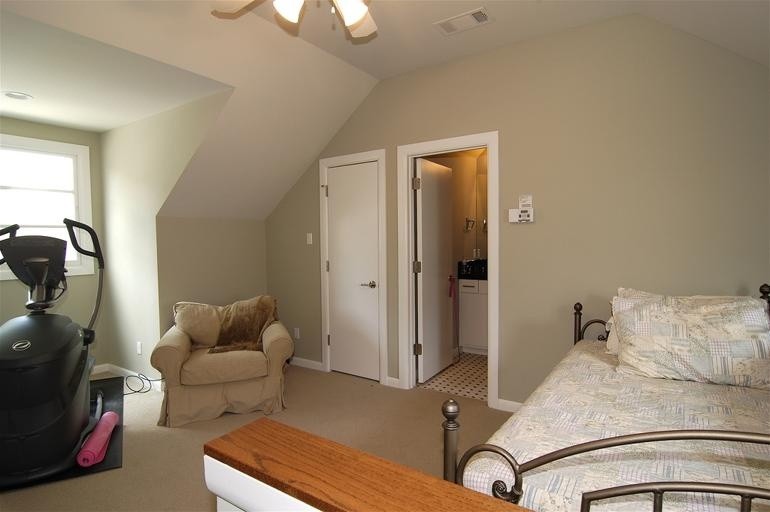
[441,284,770,510]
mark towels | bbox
[477,211,488,242]
[75,410,119,467]
[460,216,476,239]
[207,294,296,372]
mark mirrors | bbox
[475,146,487,258]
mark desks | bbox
[199,414,536,511]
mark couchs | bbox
[150,295,296,428]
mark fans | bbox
[213,1,379,42]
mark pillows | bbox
[611,297,770,392]
[606,286,646,357]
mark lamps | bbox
[271,1,369,31]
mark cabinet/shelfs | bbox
[457,279,488,356]
